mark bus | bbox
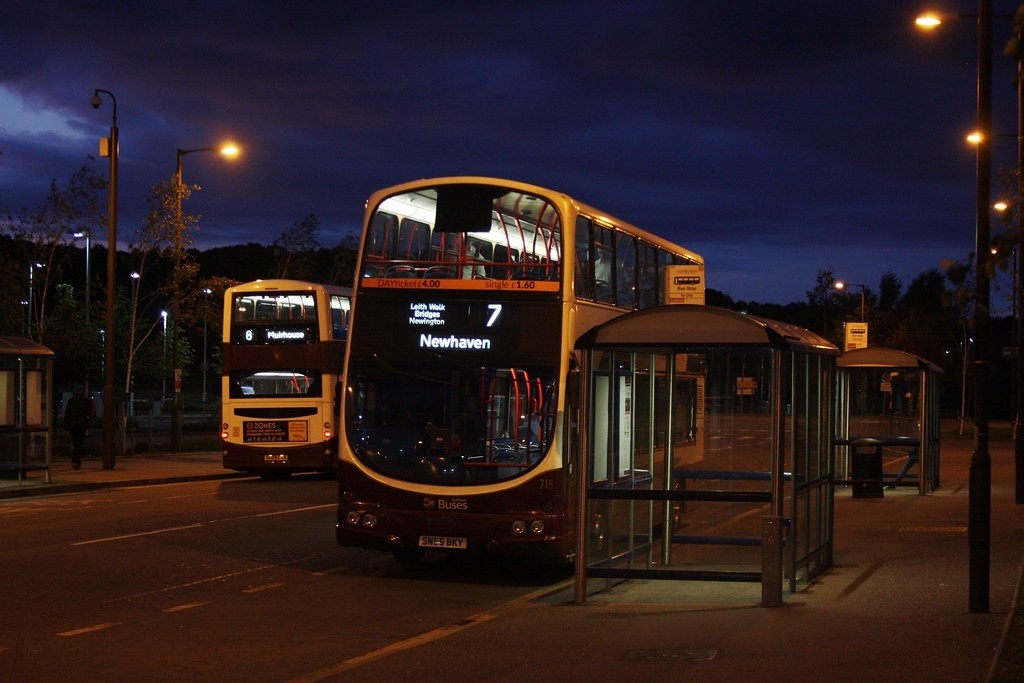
[219,280,353,480]
[337,175,705,582]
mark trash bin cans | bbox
[787,404,792,415]
[850,438,885,499]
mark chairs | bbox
[486,441,541,479]
[595,279,612,303]
[383,265,418,278]
[423,266,458,280]
[510,270,546,281]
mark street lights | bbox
[915,0,1024,615]
[169,141,241,454]
[100,329,105,371]
[28,263,43,332]
[834,282,865,324]
[161,310,168,399]
[88,87,119,470]
[202,288,212,402]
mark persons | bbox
[62,387,93,470]
[519,252,531,271]
[594,248,611,303]
[517,397,539,443]
[469,242,486,278]
